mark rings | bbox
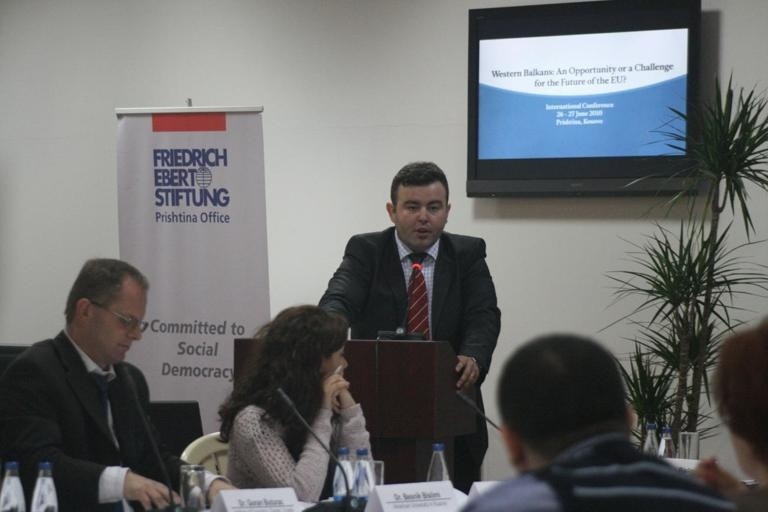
[469,374,475,379]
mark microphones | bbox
[119,357,175,512]
[455,391,502,432]
[275,388,365,512]
[377,264,422,340]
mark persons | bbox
[694,314,768,512]
[457,331,737,512]
[317,162,502,497]
[217,305,376,504]
[0,257,237,512]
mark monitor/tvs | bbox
[467,0,701,197]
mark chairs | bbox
[178,430,232,478]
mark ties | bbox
[92,373,118,466]
[405,253,431,341]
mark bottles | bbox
[30,461,59,512]
[427,444,450,480]
[655,426,676,459]
[643,422,659,459]
[0,460,27,512]
[332,445,375,511]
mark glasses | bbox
[91,302,148,334]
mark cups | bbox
[678,429,700,457]
[179,463,207,511]
